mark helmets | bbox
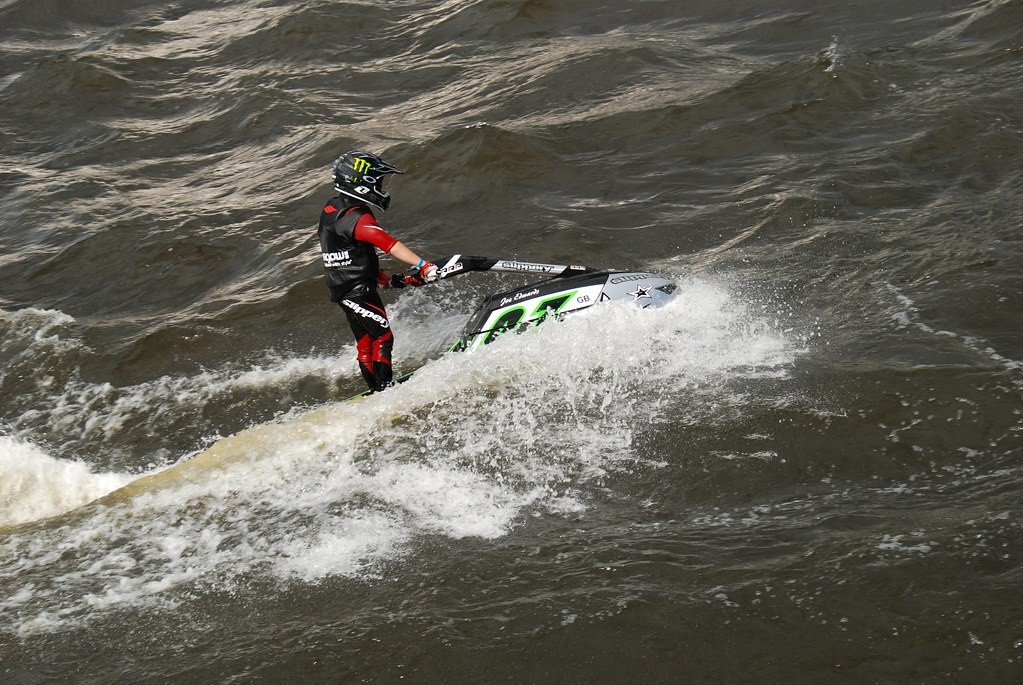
[333,150,404,213]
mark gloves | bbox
[419,260,442,284]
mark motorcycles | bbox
[335,253,684,405]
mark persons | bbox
[318,151,440,398]
